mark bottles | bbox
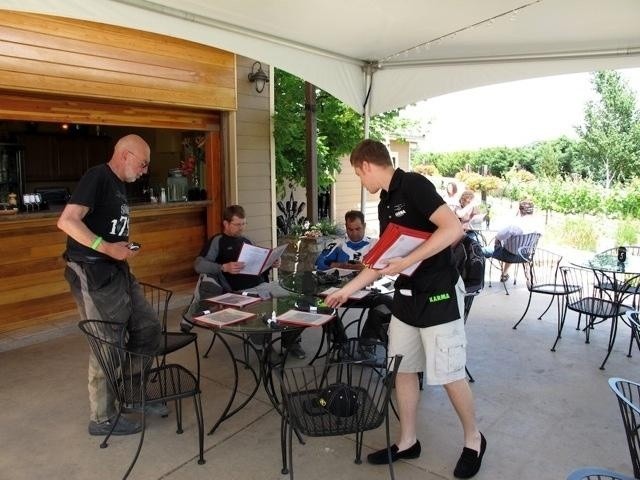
[142,185,167,205]
[166,169,189,202]
[484,207,494,229]
[618,247,625,261]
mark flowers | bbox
[178,135,205,187]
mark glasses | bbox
[128,151,150,168]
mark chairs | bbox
[271,354,402,480]
[75,282,205,479]
[34,186,71,205]
[566,377,640,480]
[485,227,639,369]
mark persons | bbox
[450,189,482,243]
[56,132,175,437]
[494,199,542,290]
[441,182,459,205]
[324,136,487,480]
[312,209,394,364]
[194,204,308,370]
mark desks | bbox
[180,267,397,444]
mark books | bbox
[360,223,435,279]
[232,240,292,277]
[277,307,337,327]
[201,289,261,309]
[319,286,373,302]
[469,213,487,224]
[192,310,258,330]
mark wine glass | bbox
[23,193,43,215]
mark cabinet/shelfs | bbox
[0,143,25,209]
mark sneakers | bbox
[291,345,305,358]
[357,344,376,361]
[267,349,281,367]
[88,417,141,434]
[367,440,421,465]
[454,432,486,479]
[120,402,171,415]
[330,341,349,361]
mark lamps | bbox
[248,61,269,93]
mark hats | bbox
[304,383,359,417]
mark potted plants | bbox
[278,218,346,273]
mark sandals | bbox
[501,274,509,281]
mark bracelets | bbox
[89,235,105,250]
